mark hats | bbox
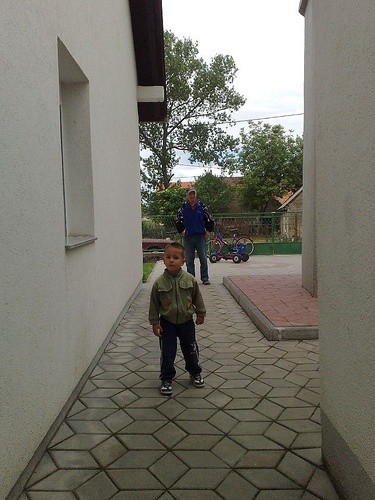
[186,188,196,194]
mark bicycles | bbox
[205,226,255,258]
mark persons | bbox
[149,242,207,395]
[175,187,215,285]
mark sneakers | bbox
[204,279,210,285]
[157,381,173,394]
[190,373,205,387]
